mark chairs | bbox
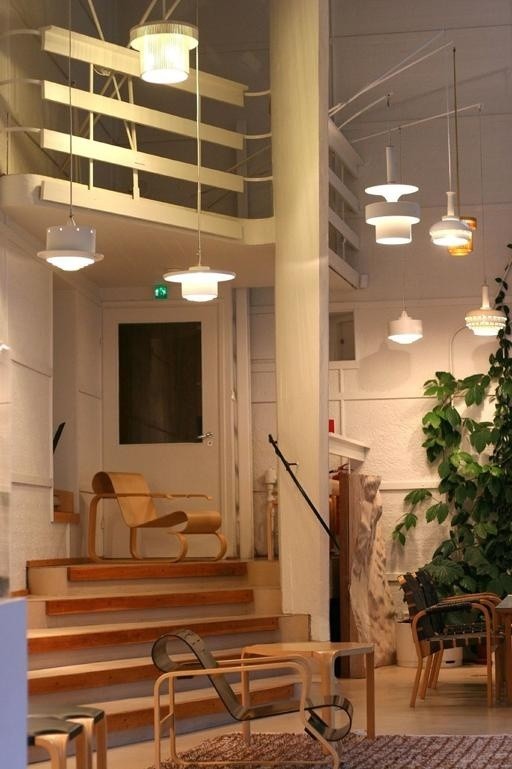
[151,628,353,769]
[88,471,228,563]
[397,567,502,708]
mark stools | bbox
[25,704,107,769]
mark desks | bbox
[495,594,512,706]
[241,641,375,755]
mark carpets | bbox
[148,732,512,769]
